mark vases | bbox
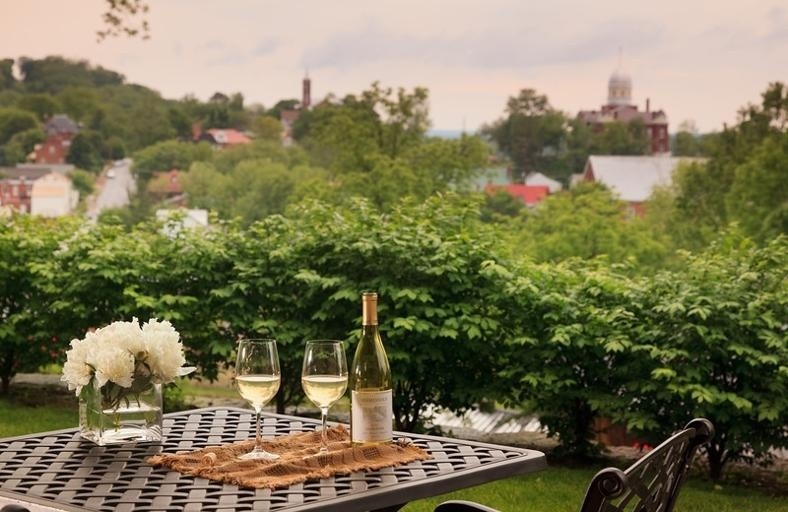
[78,385,163,446]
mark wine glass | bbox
[302,340,347,459]
[234,338,282,460]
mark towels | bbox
[146,425,432,491]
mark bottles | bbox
[350,291,394,447]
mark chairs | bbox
[434,418,715,511]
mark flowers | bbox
[60,317,197,397]
